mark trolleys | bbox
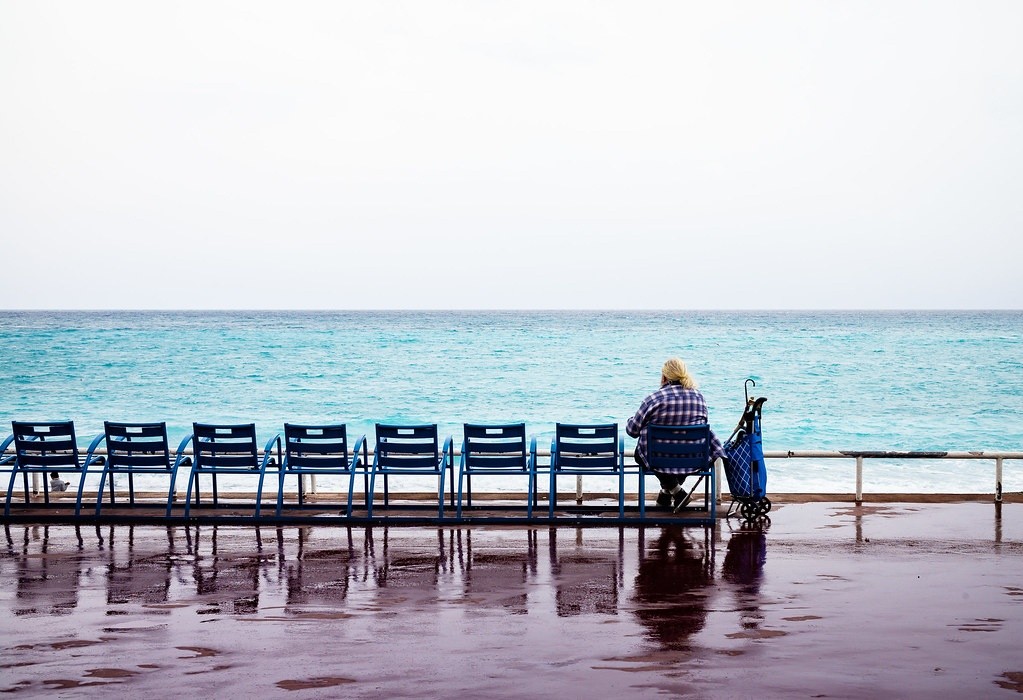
[721,398,772,522]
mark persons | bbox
[624,356,729,507]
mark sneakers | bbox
[656,491,672,507]
[673,488,693,509]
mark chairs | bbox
[638,423,715,522]
[276,422,369,527]
[368,422,455,527]
[456,423,538,527]
[94,420,199,529]
[184,422,284,524]
[5,419,116,526]
[549,422,625,525]
